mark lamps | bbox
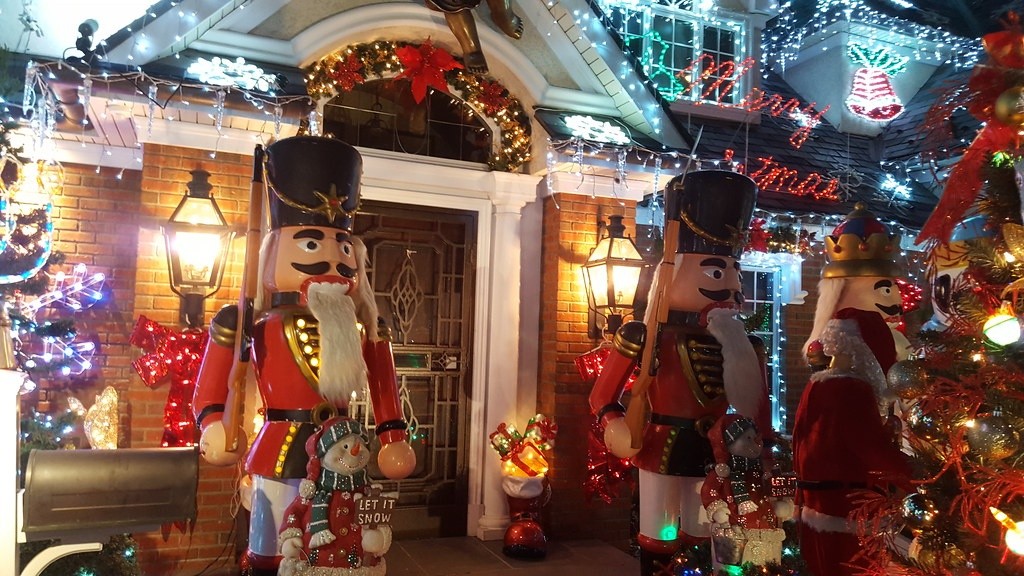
[160,166,247,331]
[585,215,644,340]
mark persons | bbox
[191,136,416,576]
[800,199,917,376]
[588,168,772,576]
[792,309,924,575]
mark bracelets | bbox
[597,403,626,424]
[375,419,408,437]
[196,404,226,430]
